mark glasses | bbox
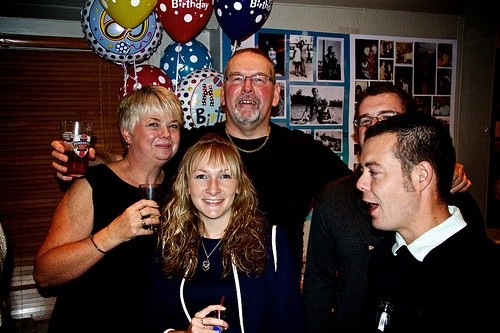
[223,72,275,86]
[352,111,400,127]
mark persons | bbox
[33,49,500,333]
[264,38,451,169]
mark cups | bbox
[59,120,93,177]
[139,184,161,230]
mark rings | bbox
[139,211,143,217]
[201,317,204,325]
[141,219,146,226]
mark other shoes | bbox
[301,74,307,78]
[296,75,299,76]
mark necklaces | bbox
[226,129,271,152]
[201,238,222,271]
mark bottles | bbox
[370,301,394,333]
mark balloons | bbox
[81,0,273,130]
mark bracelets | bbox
[90,234,107,254]
